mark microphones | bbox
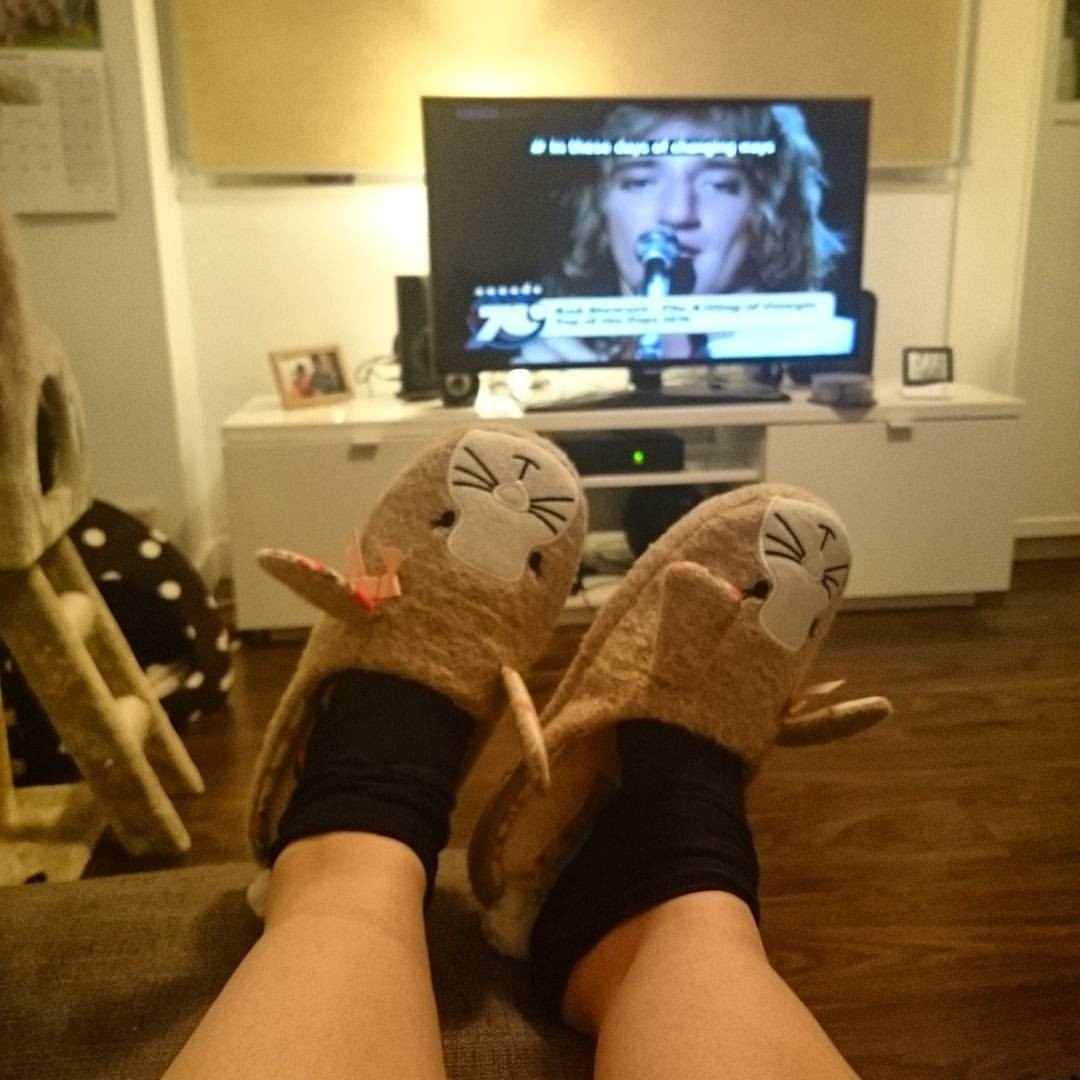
[635,228,677,353]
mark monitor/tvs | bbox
[419,97,875,371]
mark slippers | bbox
[467,484,892,959]
[242,423,588,920]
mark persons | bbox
[153,424,905,1079]
[512,95,852,360]
[295,363,313,399]
[308,354,341,394]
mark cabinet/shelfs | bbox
[216,367,1030,616]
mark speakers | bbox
[439,373,479,407]
[791,288,875,381]
[396,275,438,398]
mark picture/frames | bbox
[899,345,955,388]
[265,344,357,408]
